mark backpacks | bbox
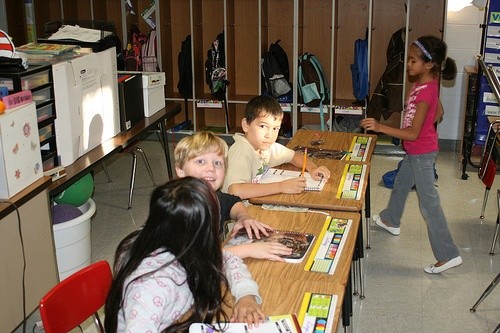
[122,24,160,72]
[350,27,369,102]
[206,30,226,101]
[177,35,193,99]
[298,52,330,107]
[261,40,293,103]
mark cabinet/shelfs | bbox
[5,0,449,139]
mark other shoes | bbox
[372,215,400,235]
[424,256,462,273]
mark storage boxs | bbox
[0,27,167,200]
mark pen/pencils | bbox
[302,147,307,177]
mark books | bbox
[15,42,79,65]
[258,167,326,191]
[482,92,500,116]
[224,226,316,262]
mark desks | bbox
[196,208,361,333]
[0,177,60,333]
[50,106,174,244]
[249,131,380,299]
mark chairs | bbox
[470,121,500,333]
[33,260,113,333]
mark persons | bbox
[104,175,266,333]
[173,130,292,262]
[221,93,330,199]
[360,35,463,274]
